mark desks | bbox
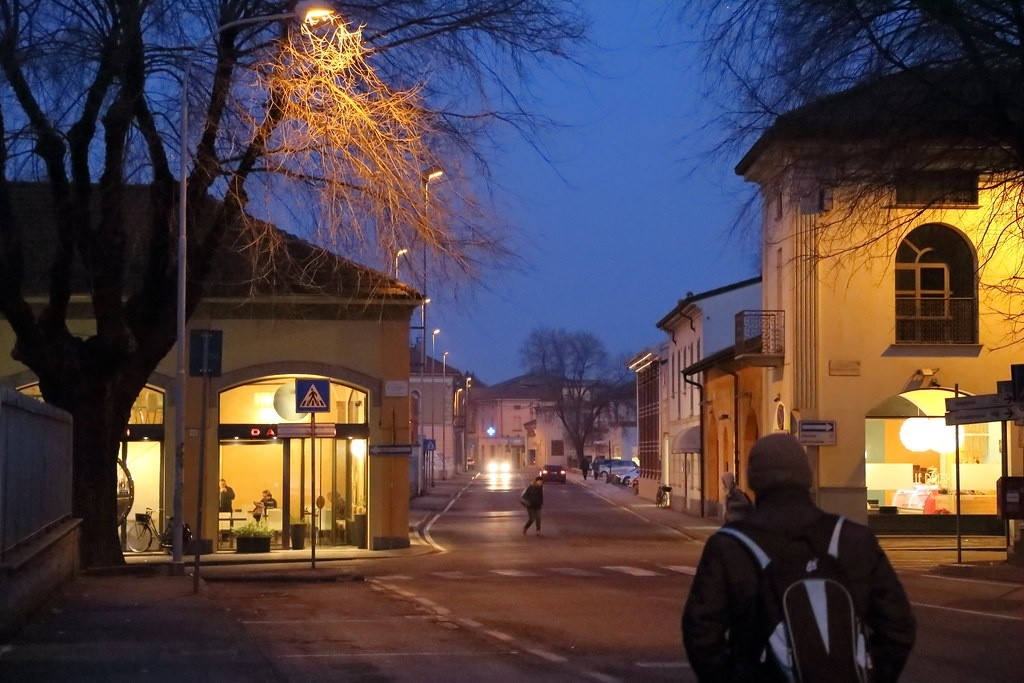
[936,493,997,514]
[218,518,247,551]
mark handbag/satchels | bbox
[520,495,531,508]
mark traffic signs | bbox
[945,405,1022,425]
[945,392,1021,411]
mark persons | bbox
[253,490,277,521]
[520,477,543,535]
[219,479,235,542]
[681,435,915,683]
[591,459,599,480]
[581,458,588,480]
[722,472,749,520]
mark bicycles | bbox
[656,482,673,508]
[127,507,193,555]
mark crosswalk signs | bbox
[296,378,330,412]
[423,439,437,451]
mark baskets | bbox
[135,513,151,523]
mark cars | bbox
[539,464,566,483]
[588,458,640,488]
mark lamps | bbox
[719,414,730,420]
[917,368,933,376]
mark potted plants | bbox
[354,505,367,549]
[290,515,312,550]
[230,521,275,553]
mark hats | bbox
[747,433,811,492]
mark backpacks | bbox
[716,511,875,683]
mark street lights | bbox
[395,248,409,281]
[417,297,432,496]
[430,328,440,486]
[442,352,449,482]
[464,377,471,473]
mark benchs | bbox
[336,519,346,543]
